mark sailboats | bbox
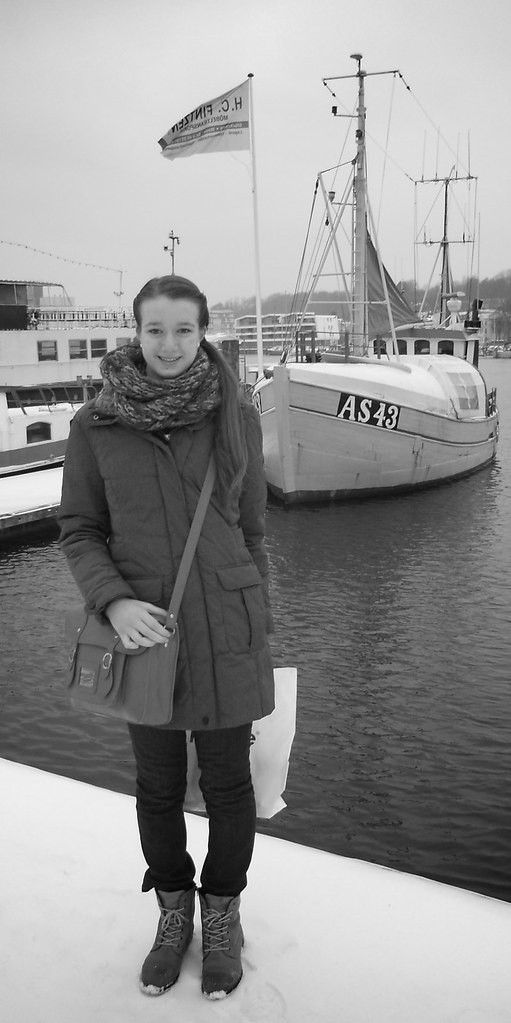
[249,50,506,504]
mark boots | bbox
[140,880,197,994]
[197,887,244,998]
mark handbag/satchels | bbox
[183,667,298,819]
[64,605,178,727]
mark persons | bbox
[57,276,276,1001]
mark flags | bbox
[157,77,252,161]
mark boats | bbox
[0,275,247,531]
